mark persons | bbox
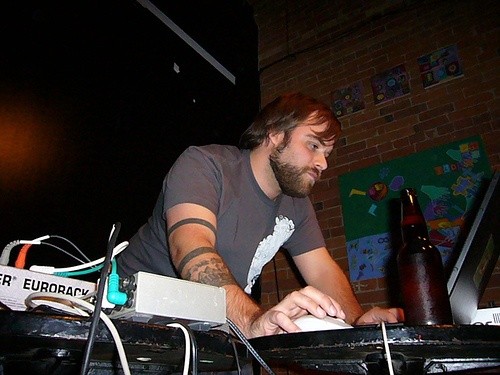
[110,90,407,340]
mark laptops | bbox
[355,171,500,330]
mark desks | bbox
[0,310,500,375]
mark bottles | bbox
[396,188,453,325]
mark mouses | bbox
[277,315,354,334]
